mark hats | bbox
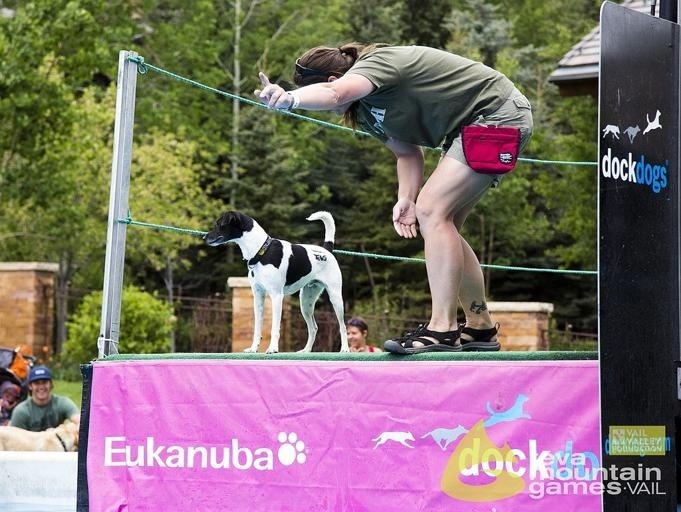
[27,367,52,384]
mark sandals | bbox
[384,322,501,354]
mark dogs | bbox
[0,419,79,452]
[201,210,350,355]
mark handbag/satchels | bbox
[462,125,521,174]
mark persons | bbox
[254,42,534,355]
[346,317,381,353]
[12,368,79,429]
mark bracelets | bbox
[287,91,300,109]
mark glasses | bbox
[348,319,367,329]
[295,58,343,78]
